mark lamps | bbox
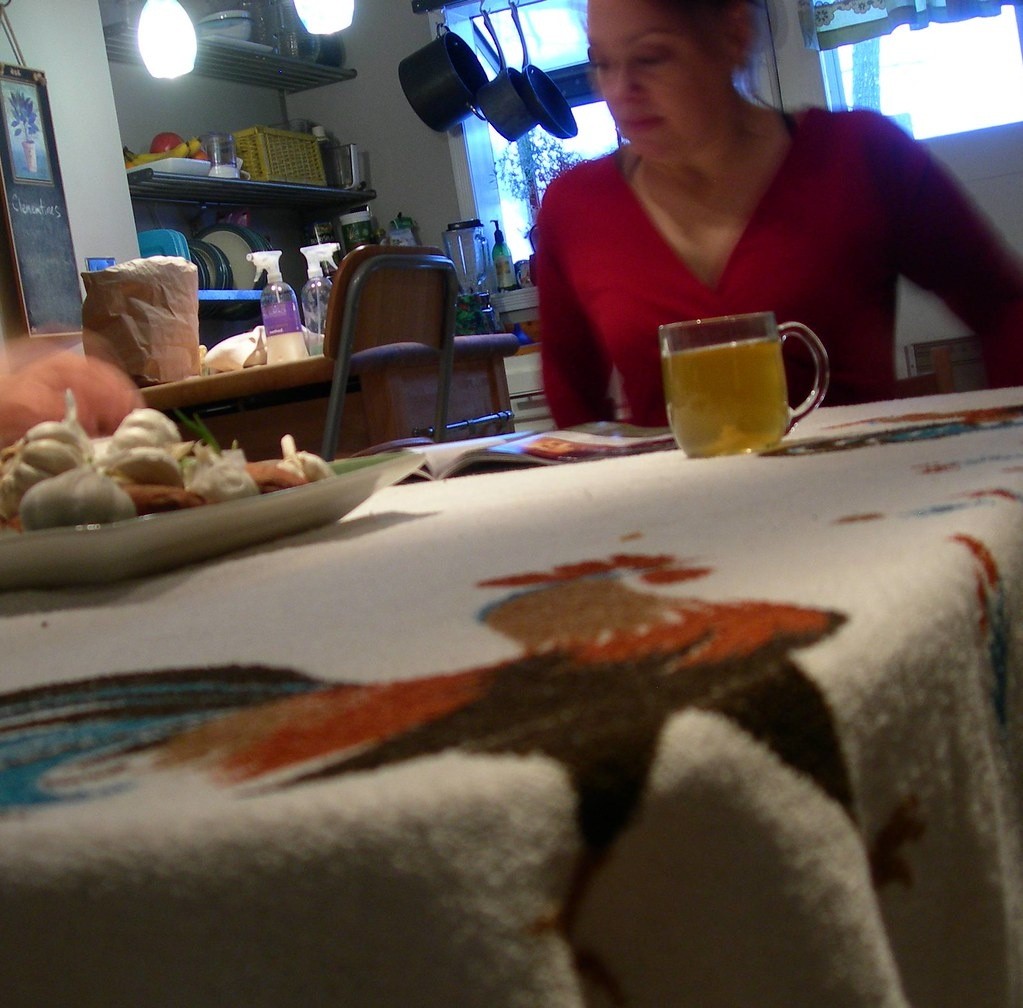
[293,0,355,35]
[137,0,199,79]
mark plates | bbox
[0,451,428,589]
[137,229,191,263]
[188,221,273,290]
[125,157,211,177]
[198,33,273,52]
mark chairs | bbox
[318,245,458,463]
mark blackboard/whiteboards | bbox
[0,64,87,339]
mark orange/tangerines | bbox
[125,161,135,169]
[188,150,209,161]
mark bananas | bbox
[123,136,202,166]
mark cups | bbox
[658,310,830,458]
[209,135,235,165]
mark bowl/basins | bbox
[194,10,255,41]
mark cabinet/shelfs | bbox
[105,18,378,210]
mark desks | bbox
[22,387,1023,1008]
[492,286,538,332]
[143,330,522,460]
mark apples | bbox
[150,132,184,153]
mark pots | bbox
[509,2,578,138]
[399,23,490,133]
[476,10,540,142]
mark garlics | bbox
[0,408,336,532]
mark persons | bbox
[535,0,1023,428]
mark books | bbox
[403,429,677,481]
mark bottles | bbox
[272,5,297,57]
[490,219,518,292]
[312,125,344,188]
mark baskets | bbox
[230,124,328,186]
